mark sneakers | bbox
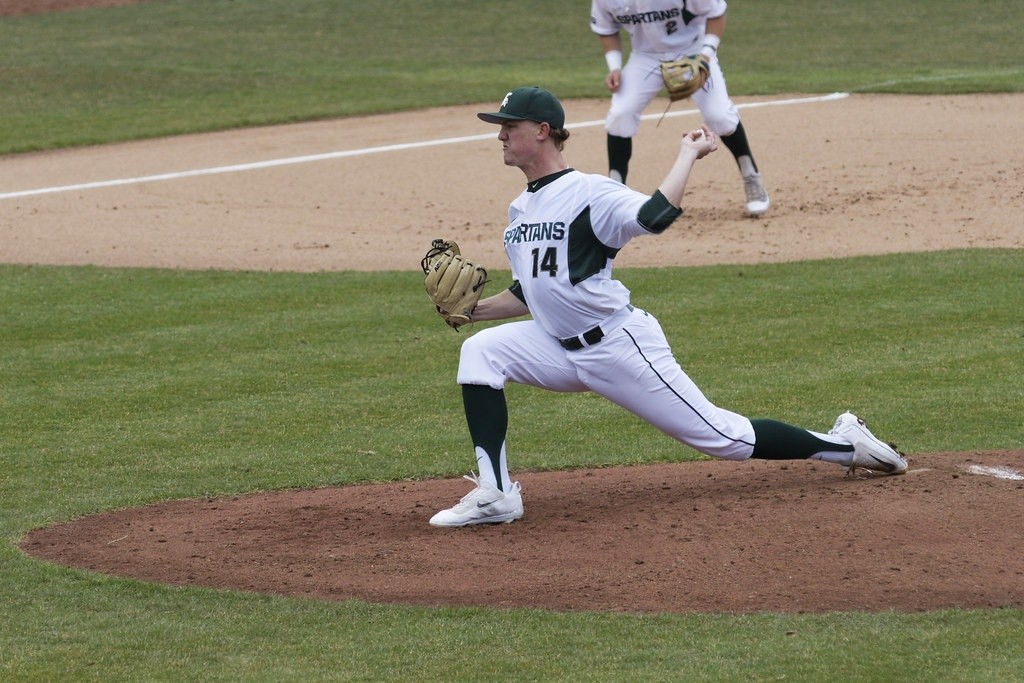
[828,413,908,480]
[429,470,523,527]
[743,173,769,217]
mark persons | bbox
[420,84,908,528]
[587,0,769,215]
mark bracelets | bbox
[701,34,720,59]
[604,50,622,70]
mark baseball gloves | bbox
[658,56,712,101]
[421,238,489,331]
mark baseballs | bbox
[692,127,711,143]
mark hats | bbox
[477,86,565,129]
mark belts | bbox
[558,304,634,349]
